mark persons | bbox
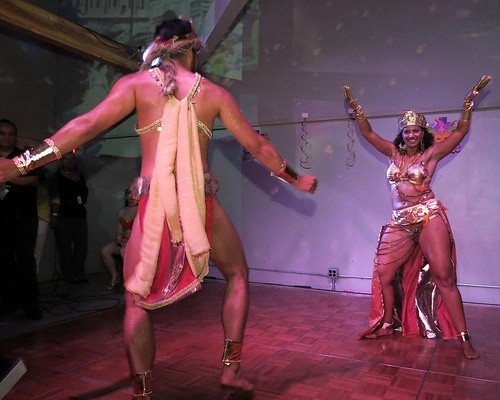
[101,187,138,290]
[0,118,88,321]
[343,75,491,359]
[0,18,319,400]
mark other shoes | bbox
[26,303,44,322]
[107,277,122,291]
